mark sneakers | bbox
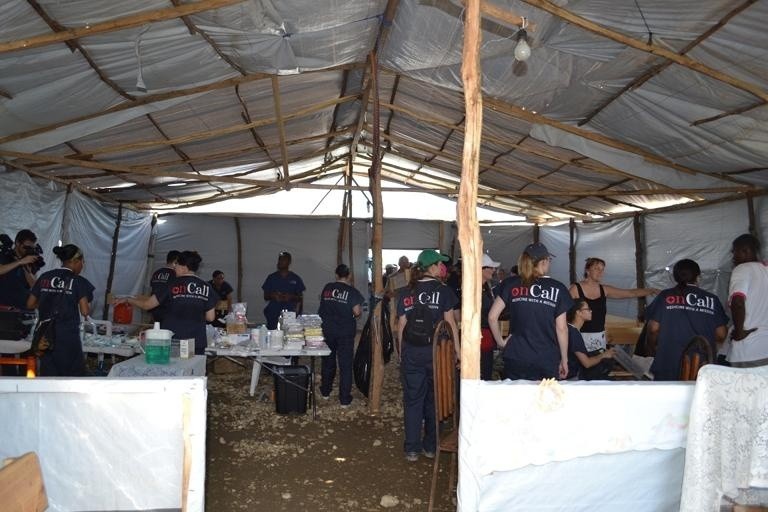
[423,448,435,458]
[407,452,419,461]
[321,391,330,397]
[341,396,353,405]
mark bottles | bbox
[258,324,268,349]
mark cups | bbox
[139,330,173,365]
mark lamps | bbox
[513,27,532,61]
[134,22,152,94]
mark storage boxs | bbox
[272,363,311,415]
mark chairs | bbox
[427,320,460,512]
[79,319,115,376]
[0,308,40,376]
[678,335,715,381]
[0,355,37,379]
[678,363,768,512]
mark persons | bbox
[481,253,501,379]
[441,251,463,295]
[487,243,568,380]
[719,234,768,368]
[567,257,660,352]
[632,322,653,378]
[150,249,181,326]
[112,250,217,355]
[565,300,618,380]
[641,262,727,380]
[398,250,461,461]
[261,251,306,331]
[27,245,95,377]
[205,271,234,326]
[381,258,413,352]
[319,265,364,406]
[0,230,46,341]
[501,265,518,285]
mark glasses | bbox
[581,307,591,312]
[173,262,178,267]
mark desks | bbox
[21,317,142,336]
[79,332,144,377]
[106,353,207,377]
[497,314,645,353]
[204,341,332,423]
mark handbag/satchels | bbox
[481,329,496,352]
[31,273,75,357]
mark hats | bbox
[523,242,556,260]
[482,254,501,268]
[386,264,397,269]
[418,249,449,267]
